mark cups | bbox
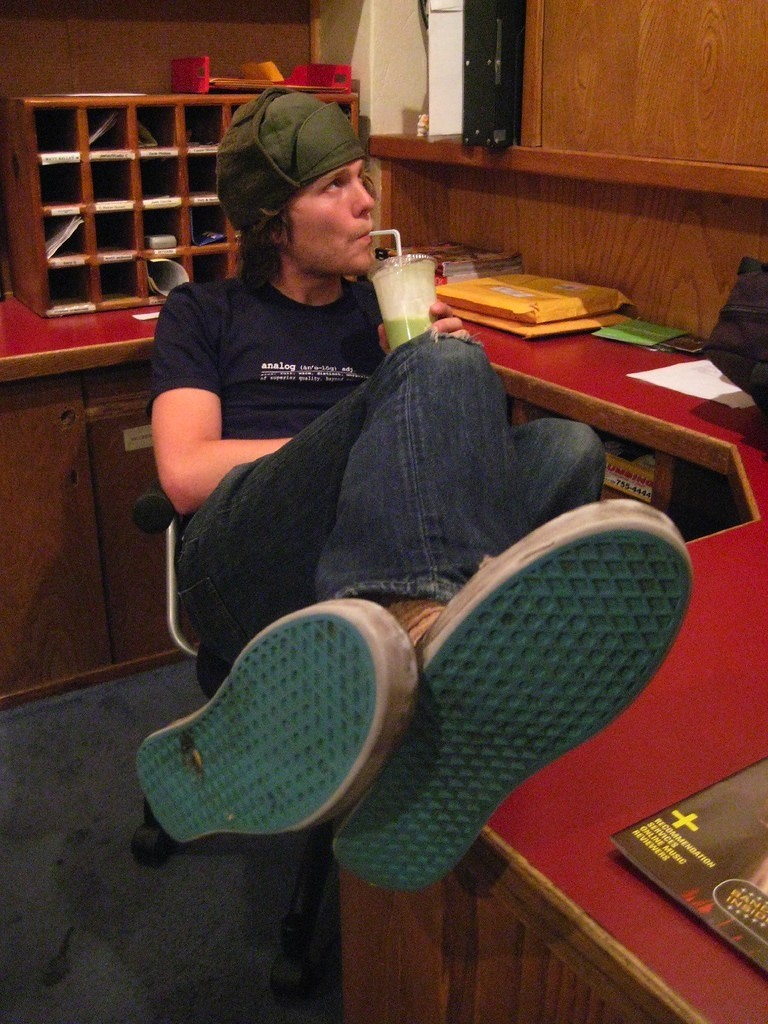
[367,254,438,349]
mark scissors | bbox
[375,248,397,261]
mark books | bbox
[611,756,768,976]
[375,240,523,286]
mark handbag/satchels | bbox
[702,256,768,421]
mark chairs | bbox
[129,345,333,1002]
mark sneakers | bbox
[136,602,420,844]
[333,499,694,891]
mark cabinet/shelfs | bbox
[0,94,360,320]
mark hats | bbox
[215,86,365,230]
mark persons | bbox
[134,82,694,894]
